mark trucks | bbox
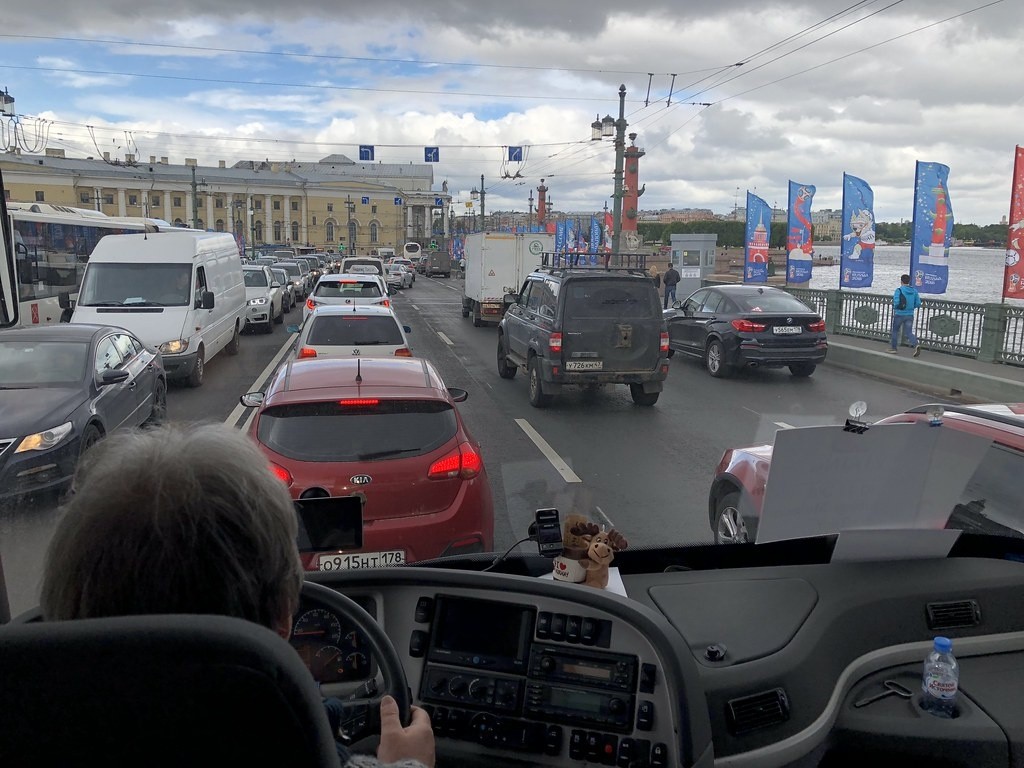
[459,229,557,328]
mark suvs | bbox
[497,252,676,409]
[705,397,1024,557]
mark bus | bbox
[0,168,208,329]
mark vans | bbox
[57,229,249,388]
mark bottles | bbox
[921,637,959,719]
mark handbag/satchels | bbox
[896,288,907,310]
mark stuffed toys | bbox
[571,522,628,588]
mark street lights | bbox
[590,84,630,272]
[470,174,487,231]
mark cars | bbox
[238,352,496,574]
[660,282,829,378]
[285,303,415,359]
[302,271,398,324]
[241,247,417,336]
[0,322,169,516]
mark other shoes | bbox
[914,344,920,357]
[662,308,667,310]
[888,349,897,353]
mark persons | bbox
[339,243,344,256]
[885,274,922,356]
[459,258,465,272]
[173,273,191,298]
[37,419,434,768]
[662,263,680,309]
[650,266,661,288]
